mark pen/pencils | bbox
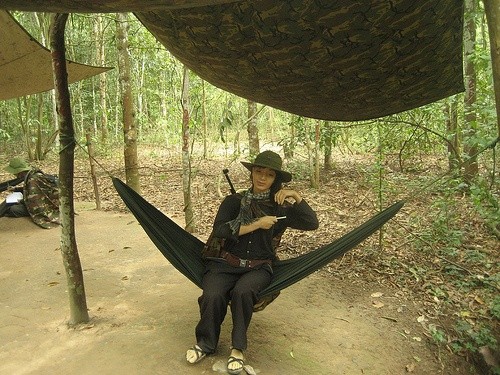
[277,215,287,220]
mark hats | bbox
[240,151,292,182]
[2,157,30,173]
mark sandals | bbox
[227,347,244,375]
[187,344,208,363]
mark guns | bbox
[222,168,236,194]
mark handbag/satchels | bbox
[200,229,242,265]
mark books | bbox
[6,192,23,203]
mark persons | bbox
[185,150,319,375]
[0,157,33,217]
